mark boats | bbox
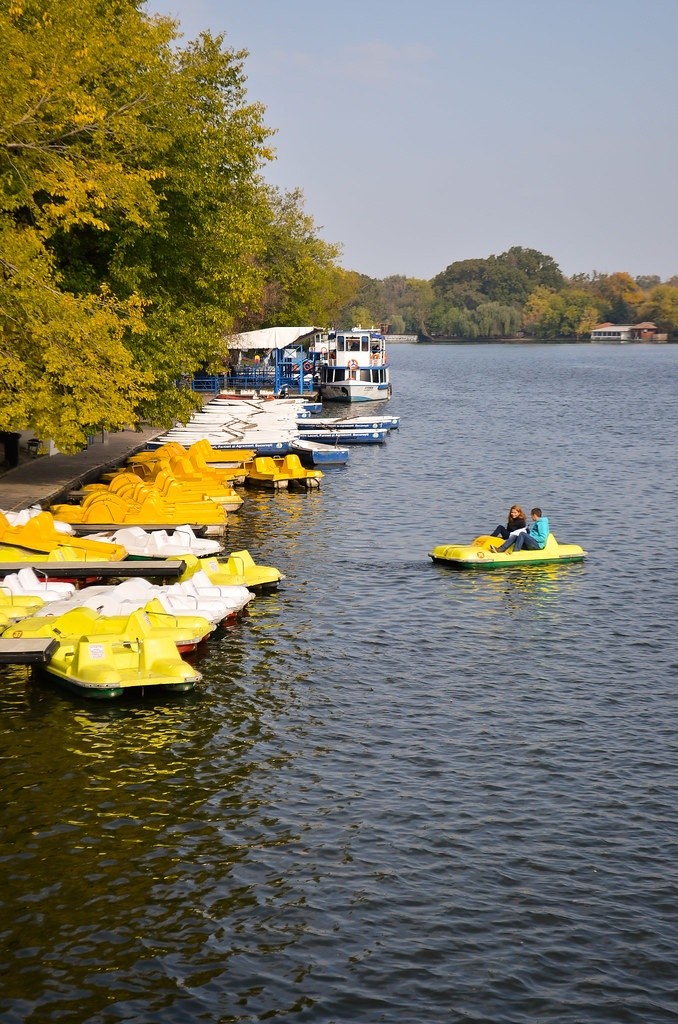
[426,532,588,571]
[320,323,392,403]
[0,398,401,700]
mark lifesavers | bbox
[371,354,379,363]
[329,352,335,359]
[348,359,358,370]
[304,362,312,370]
[321,347,327,353]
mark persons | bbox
[490,508,549,553]
[490,505,526,540]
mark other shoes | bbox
[490,545,503,553]
